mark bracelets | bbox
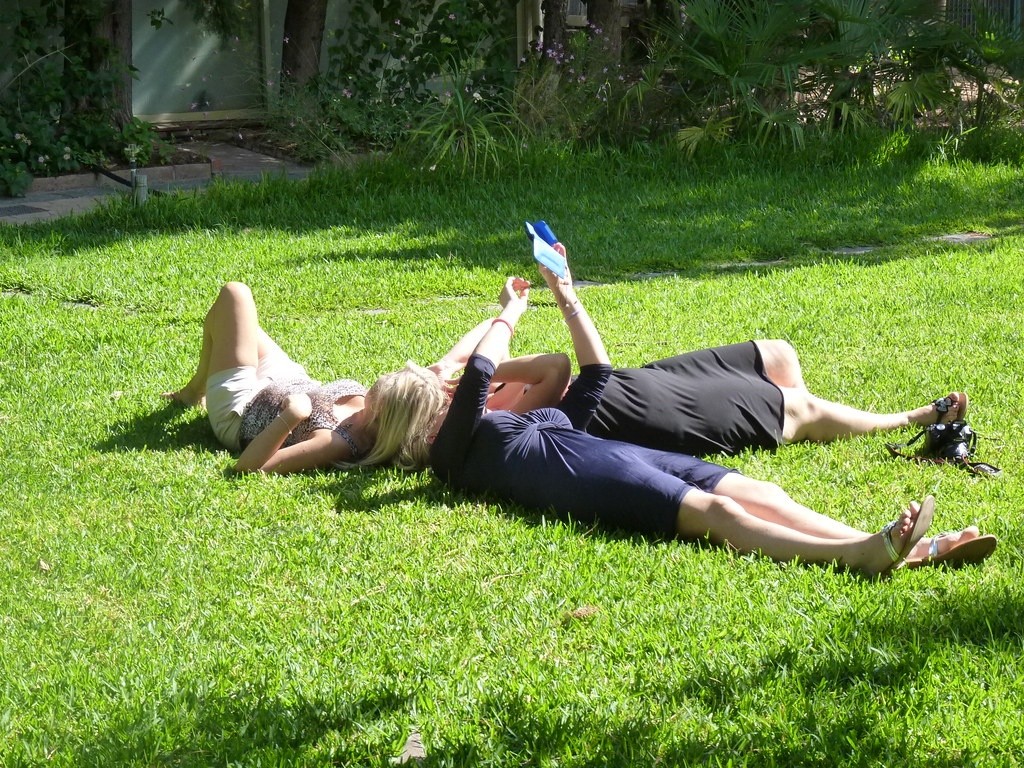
[277,415,292,434]
[492,319,513,335]
[564,304,585,322]
[558,297,579,313]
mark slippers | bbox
[881,495,997,569]
[161,390,202,408]
[923,393,968,432]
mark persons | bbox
[158,243,996,582]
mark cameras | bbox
[925,420,974,464]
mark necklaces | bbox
[343,415,354,432]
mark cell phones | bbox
[525,220,568,270]
